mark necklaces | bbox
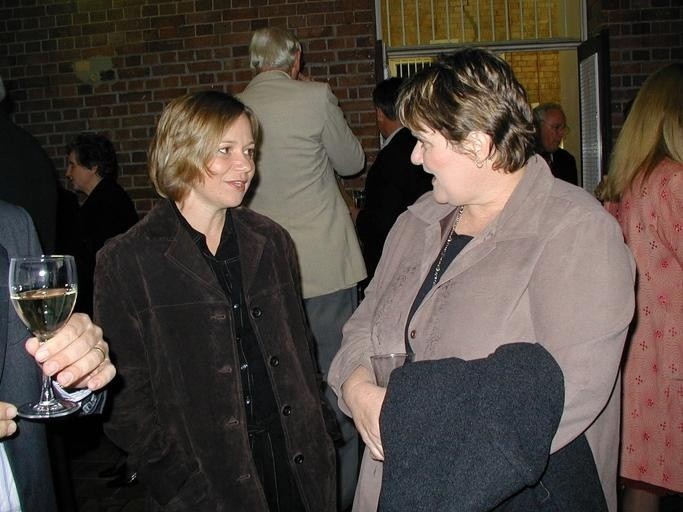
[433,205,463,288]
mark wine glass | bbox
[7,254,81,420]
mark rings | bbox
[95,347,105,359]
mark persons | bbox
[351,78,433,306]
[325,46,635,512]
[92,80,337,511]
[533,103,578,186]
[594,64,683,512]
[232,23,367,512]
[1,202,116,511]
[64,136,139,240]
